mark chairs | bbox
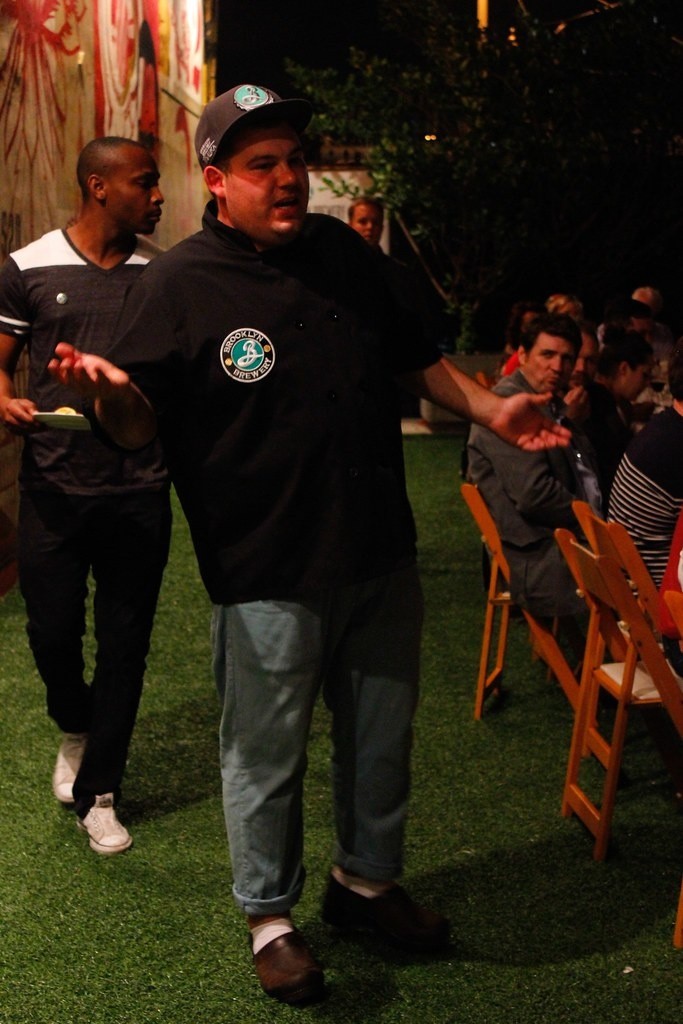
[460,481,683,863]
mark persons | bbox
[48,83,572,1000]
[608,336,683,588]
[464,312,612,616]
[500,287,673,481]
[5,136,174,853]
[348,196,384,249]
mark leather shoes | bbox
[321,874,450,953]
[249,925,324,1000]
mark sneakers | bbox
[52,732,87,801]
[76,793,133,854]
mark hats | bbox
[195,84,313,167]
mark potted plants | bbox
[279,0,615,425]
